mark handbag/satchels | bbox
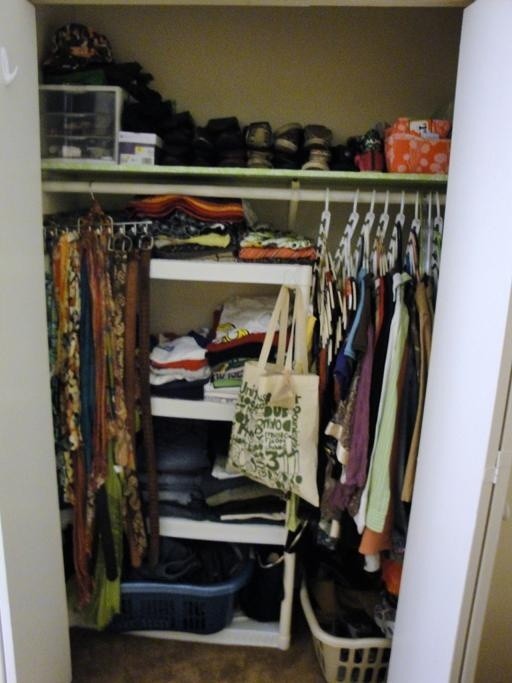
[225,284,319,508]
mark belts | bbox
[103,231,159,569]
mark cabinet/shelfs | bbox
[121,258,313,650]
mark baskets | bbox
[104,543,256,634]
[298,574,392,682]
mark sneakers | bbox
[161,110,334,170]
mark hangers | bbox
[316,237,442,365]
